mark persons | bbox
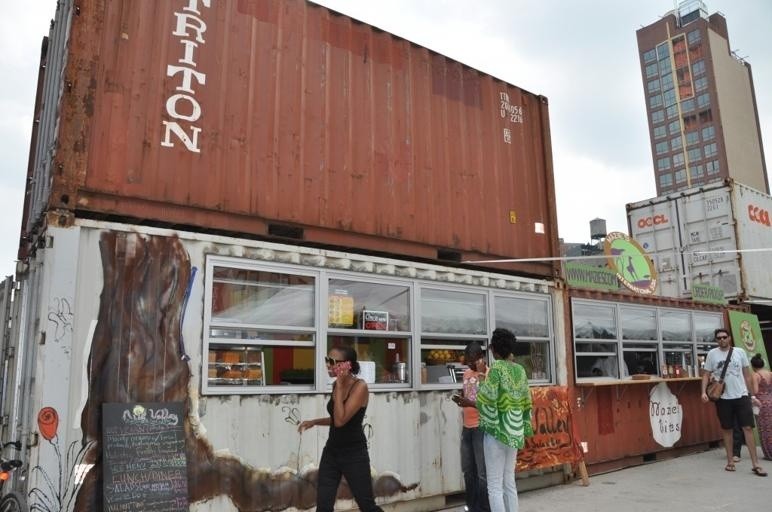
[751,353,772,461]
[297,346,385,512]
[590,344,630,379]
[701,328,769,477]
[475,328,535,512]
[450,340,491,512]
[732,413,744,461]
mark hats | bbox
[463,342,483,365]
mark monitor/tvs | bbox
[387,342,397,350]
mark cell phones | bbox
[334,361,352,375]
[453,394,459,397]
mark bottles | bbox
[750,395,760,416]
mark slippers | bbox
[752,466,768,477]
[725,463,736,472]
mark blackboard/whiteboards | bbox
[520,386,589,471]
[101,402,188,511]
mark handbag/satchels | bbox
[707,381,725,401]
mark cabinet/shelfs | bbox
[208,329,266,387]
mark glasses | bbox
[715,335,729,340]
[324,356,347,365]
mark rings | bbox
[458,402,461,405]
[341,371,343,374]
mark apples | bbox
[427,350,451,360]
